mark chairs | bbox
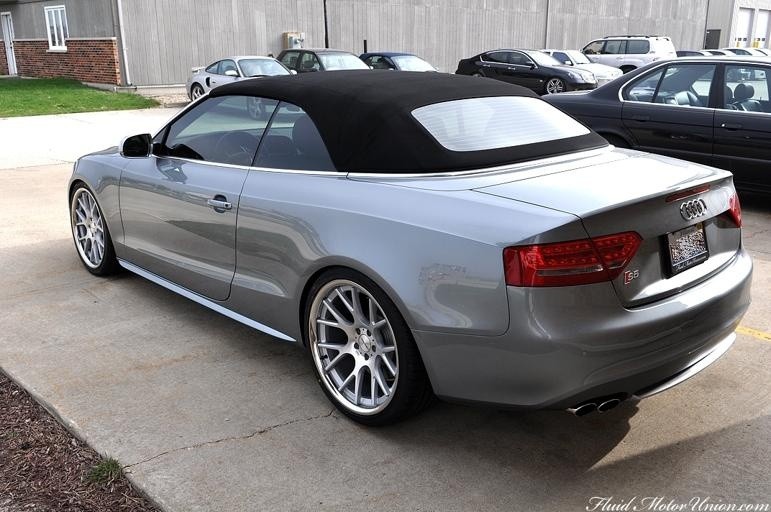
[735,83,763,113]
[664,89,705,106]
[271,114,336,171]
[723,84,737,110]
